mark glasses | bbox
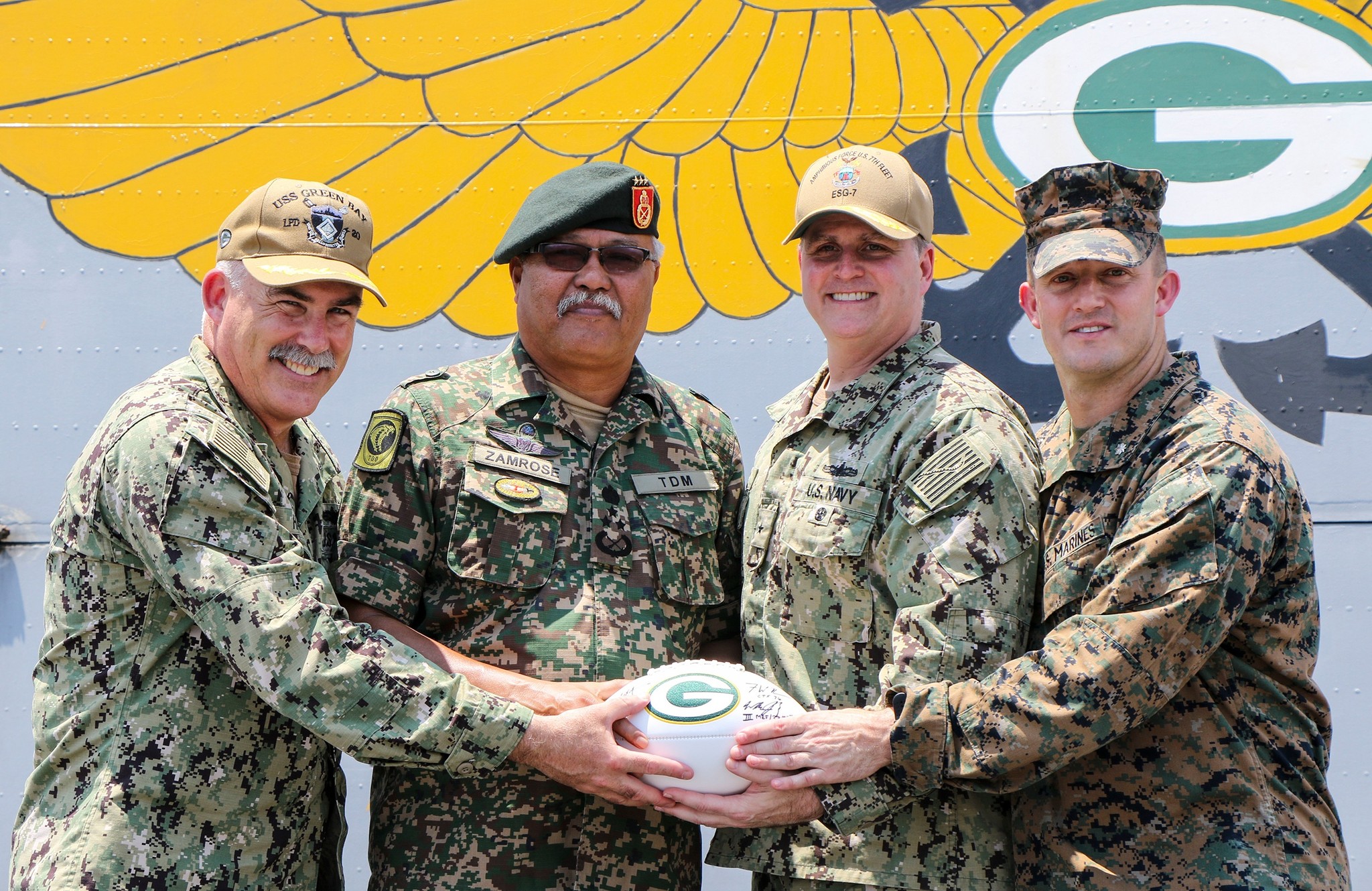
[518,241,657,274]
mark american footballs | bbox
[610,659,809,799]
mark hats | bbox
[492,160,661,266]
[1014,159,1170,280]
[781,144,934,247]
[215,177,389,309]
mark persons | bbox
[704,143,1043,891]
[727,161,1350,891]
[334,158,749,891]
[4,179,695,891]
